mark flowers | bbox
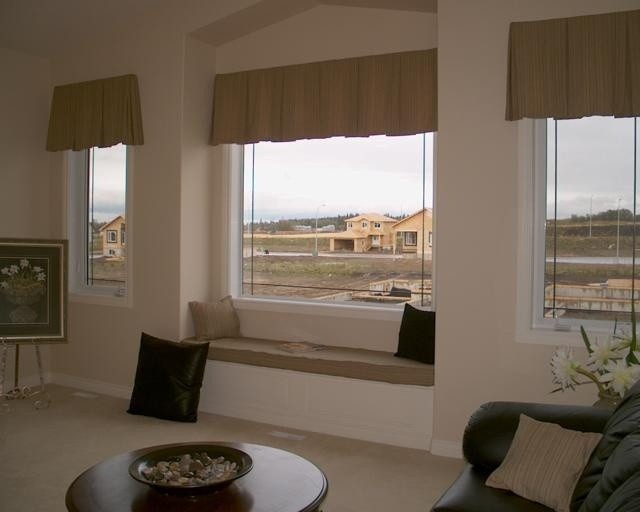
[545,319,640,396]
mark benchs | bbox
[181,337,434,452]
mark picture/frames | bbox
[0,237,69,345]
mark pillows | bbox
[126,331,210,423]
[188,294,241,341]
[484,412,606,512]
[394,303,435,365]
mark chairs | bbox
[432,381,640,512]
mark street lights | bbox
[616,196,623,265]
[589,192,596,239]
[315,202,326,256]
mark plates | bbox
[128,444,254,496]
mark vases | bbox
[592,391,624,412]
